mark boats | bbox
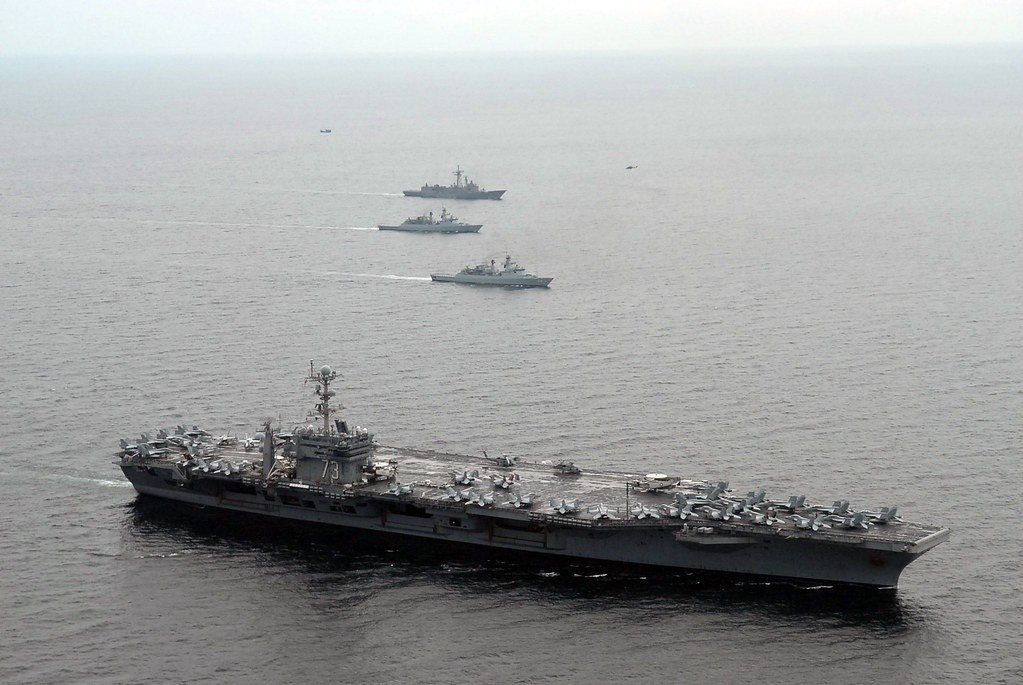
[430,247,554,287]
[111,360,951,591]
[402,164,506,201]
[378,203,483,232]
